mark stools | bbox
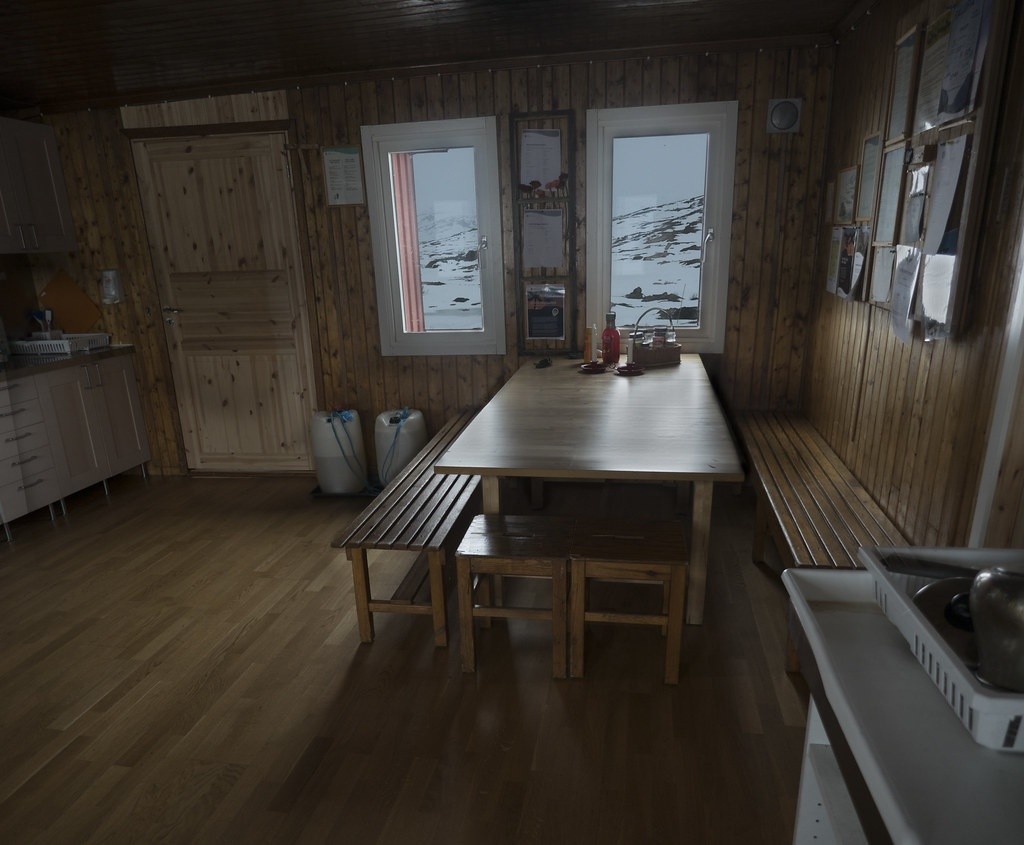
[568,516,690,686]
[454,513,579,680]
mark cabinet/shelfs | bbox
[0,117,77,254]
[0,354,152,524]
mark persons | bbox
[838,233,852,295]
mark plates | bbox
[617,365,646,376]
[580,362,609,374]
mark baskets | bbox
[10,329,111,355]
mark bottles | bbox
[627,331,646,365]
[583,322,598,363]
[638,342,652,365]
[664,329,676,346]
[601,311,621,364]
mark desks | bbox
[434,351,744,631]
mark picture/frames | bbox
[884,23,923,147]
[855,132,883,222]
[833,165,860,225]
[871,140,910,249]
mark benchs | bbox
[331,408,518,647]
[732,409,911,676]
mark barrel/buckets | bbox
[308,409,368,496]
[374,408,428,489]
